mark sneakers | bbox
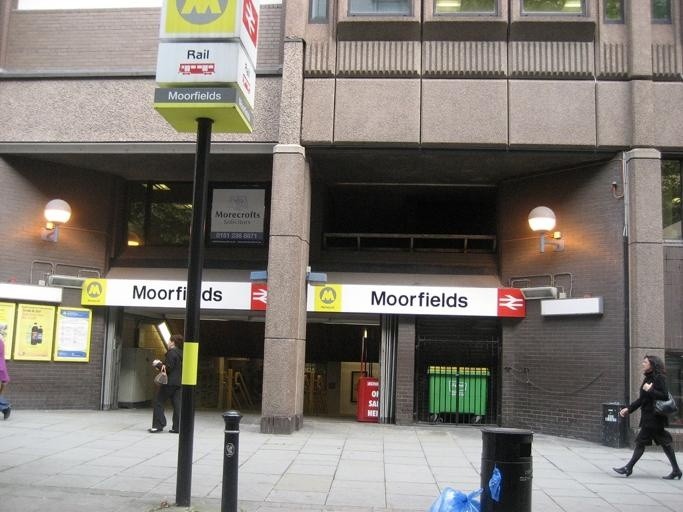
[149,427,179,433]
[4,404,12,420]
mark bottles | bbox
[31,322,44,346]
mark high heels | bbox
[613,466,632,477]
[663,470,682,480]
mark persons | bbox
[149,333,184,434]
[0,321,13,420]
[611,355,682,480]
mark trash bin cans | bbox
[481,427,533,512]
[356,377,380,423]
[601,404,626,449]
[426,367,490,424]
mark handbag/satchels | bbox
[655,392,679,415]
[154,365,168,384]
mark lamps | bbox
[527,205,564,253]
[41,197,71,242]
